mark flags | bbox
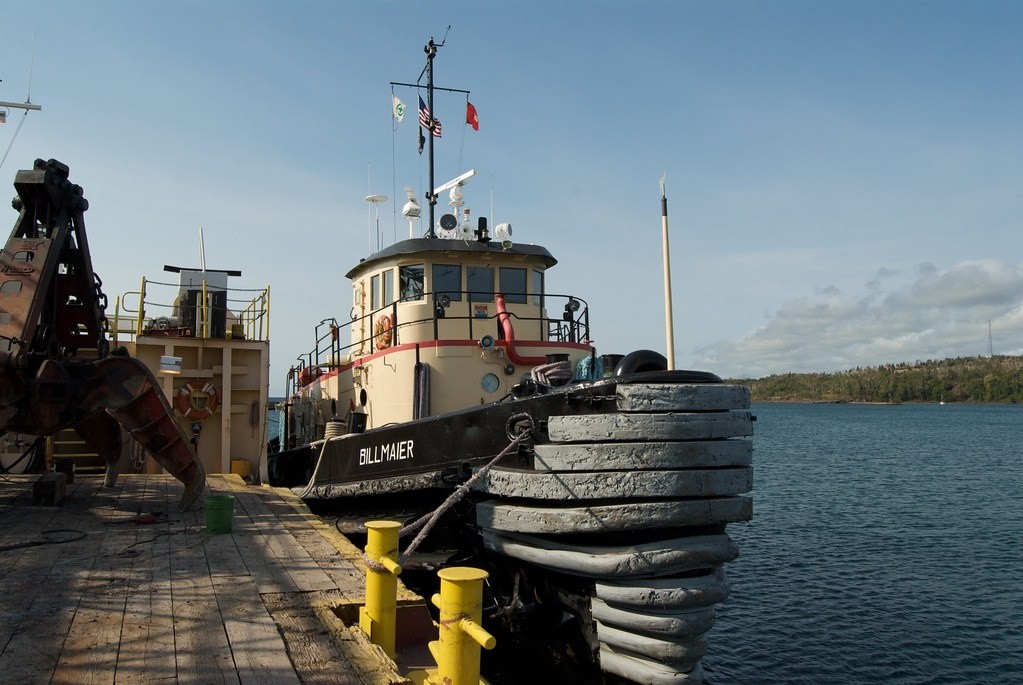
[392,95,406,123]
[418,95,442,138]
[466,102,479,131]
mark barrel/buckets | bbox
[204,495,235,534]
[601,353,626,379]
[348,411,366,432]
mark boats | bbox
[265,34,726,669]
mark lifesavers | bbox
[177,380,220,421]
[374,315,392,350]
